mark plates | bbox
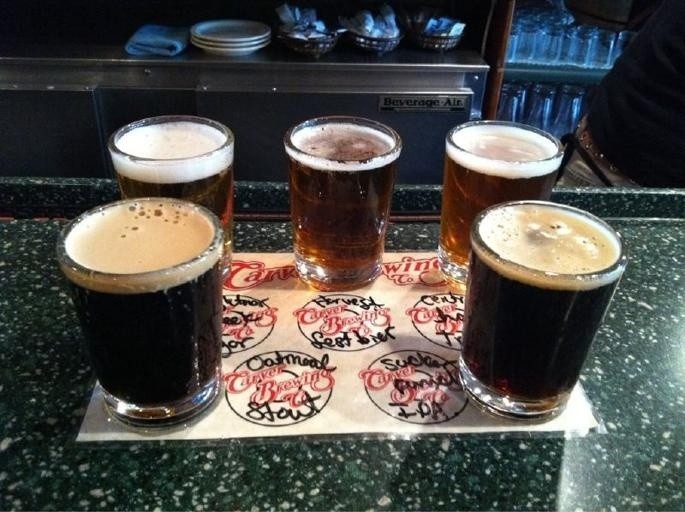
[191,19,272,57]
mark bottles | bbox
[496,82,585,135]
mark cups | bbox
[64,198,234,430]
[436,125,568,265]
[509,9,638,69]
[458,196,631,424]
[110,114,239,197]
[278,115,407,295]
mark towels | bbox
[124,11,209,58]
